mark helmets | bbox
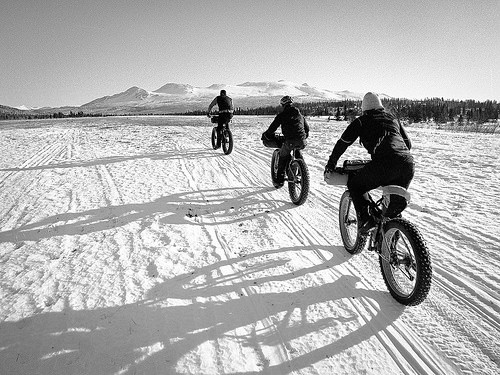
[280,95,294,104]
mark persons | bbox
[207,90,234,149]
[323,91,415,254]
[262,96,310,186]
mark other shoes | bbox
[276,178,285,186]
[358,208,375,232]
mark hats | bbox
[361,92,384,112]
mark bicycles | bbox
[263,133,310,206]
[208,115,234,155]
[325,159,432,307]
